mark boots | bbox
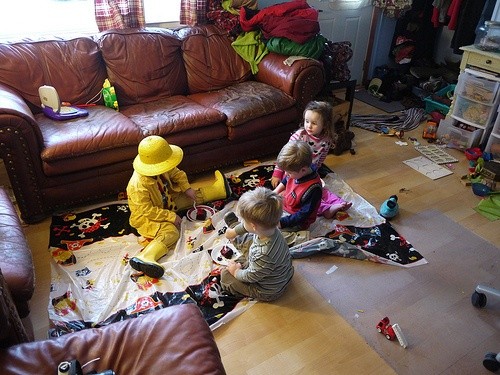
[130,239,168,279]
[199,170,230,204]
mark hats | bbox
[133,135,183,176]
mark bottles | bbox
[474,21,500,52]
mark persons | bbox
[273,140,322,232]
[271,101,353,219]
[126,135,232,277]
[220,187,294,302]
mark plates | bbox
[186,205,216,224]
[211,244,244,266]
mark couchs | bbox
[0,185,226,375]
[0,21,328,227]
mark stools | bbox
[328,78,358,129]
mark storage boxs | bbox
[323,95,350,132]
[425,84,456,115]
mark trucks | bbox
[375,316,397,341]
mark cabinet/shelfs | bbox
[438,45,500,166]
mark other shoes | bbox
[224,211,238,227]
